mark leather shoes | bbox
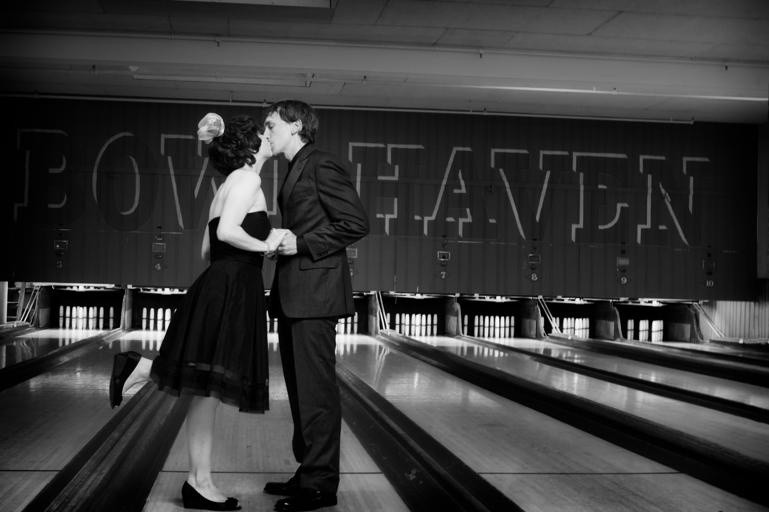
[275,488,337,512]
[264,475,300,496]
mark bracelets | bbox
[264,241,271,256]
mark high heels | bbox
[181,481,241,511]
[109,351,141,408]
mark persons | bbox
[258,99,372,511]
[108,113,294,511]
